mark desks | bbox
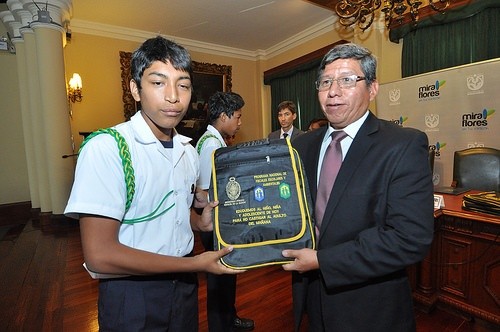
[78,131,93,139]
[407,190,500,332]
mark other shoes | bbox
[230,317,254,332]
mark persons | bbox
[194,91,254,332]
[191,43,435,332]
[308,118,328,133]
[266,101,304,142]
[62,35,247,332]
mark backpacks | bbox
[208,138,316,270]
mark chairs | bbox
[452,147,500,196]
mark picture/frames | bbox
[118,50,236,145]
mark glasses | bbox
[315,75,372,90]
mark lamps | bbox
[335,0,451,33]
[66,73,83,103]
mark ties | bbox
[314,130,348,250]
[283,133,289,139]
